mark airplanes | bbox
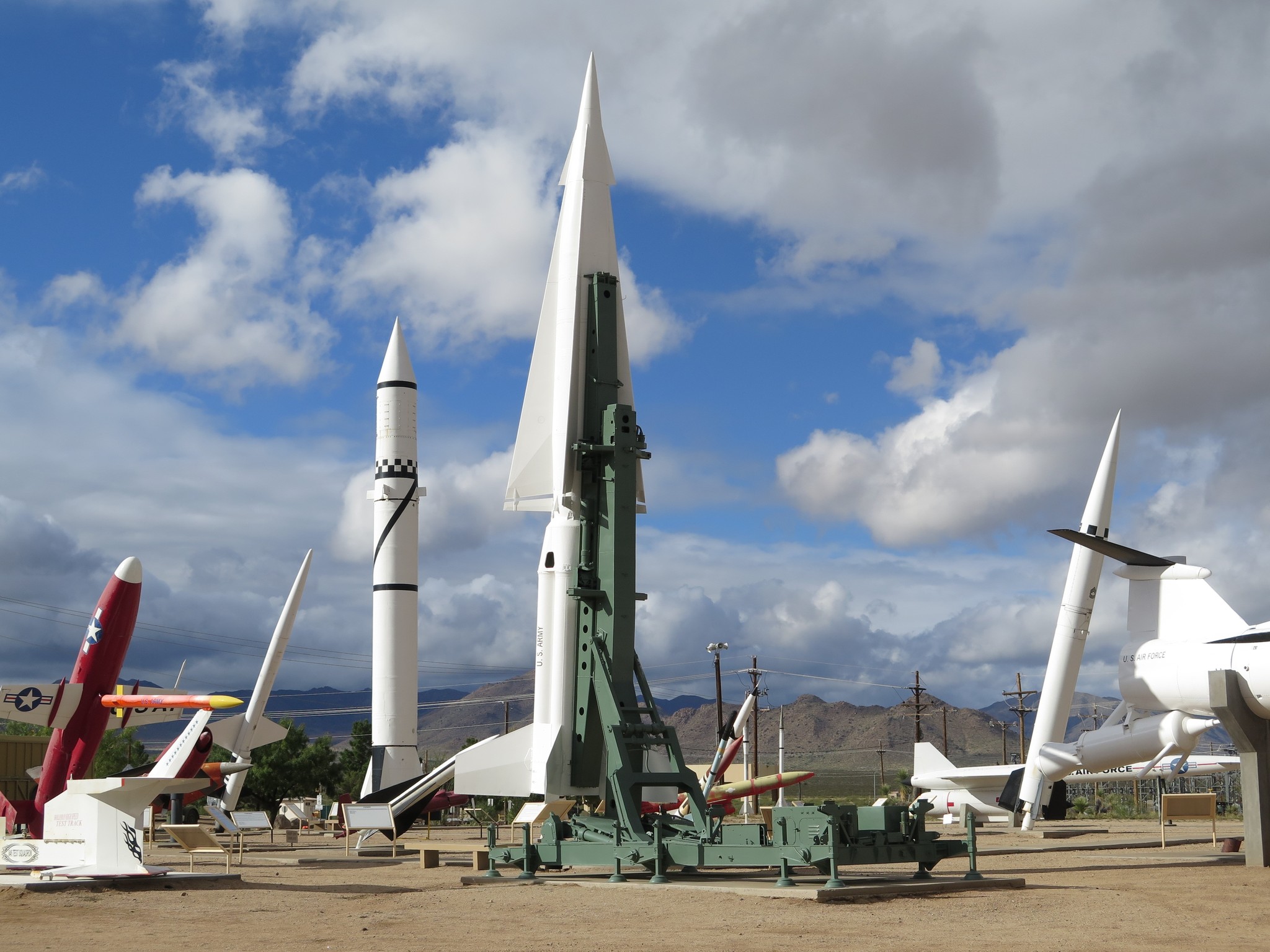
[903,738,1244,837]
[97,725,253,831]
[1032,525,1270,788]
[0,556,247,837]
